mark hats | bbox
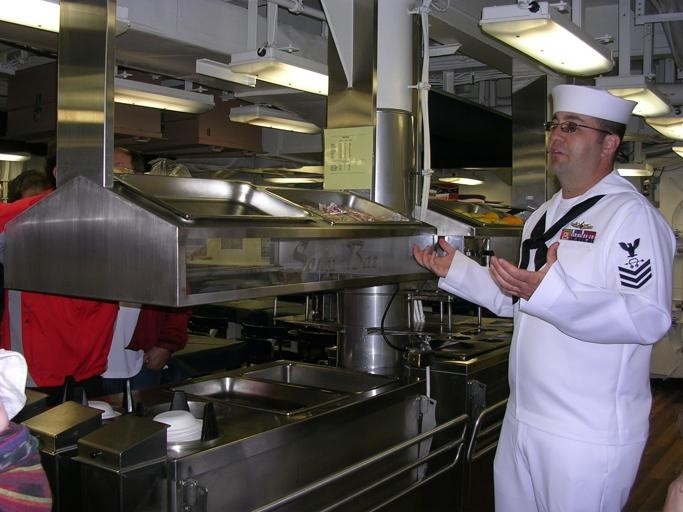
[551,84,638,125]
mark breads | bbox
[453,208,523,225]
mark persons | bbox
[662,473,682,512]
[412,83,677,512]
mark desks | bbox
[169,333,248,376]
[214,298,303,332]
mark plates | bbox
[455,192,510,210]
[154,410,203,441]
[86,400,120,419]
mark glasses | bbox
[544,121,612,135]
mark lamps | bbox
[0,1,130,41]
[256,155,325,188]
[439,169,484,187]
[112,65,214,114]
[231,1,329,97]
[0,149,33,162]
[229,98,324,136]
[480,1,682,178]
